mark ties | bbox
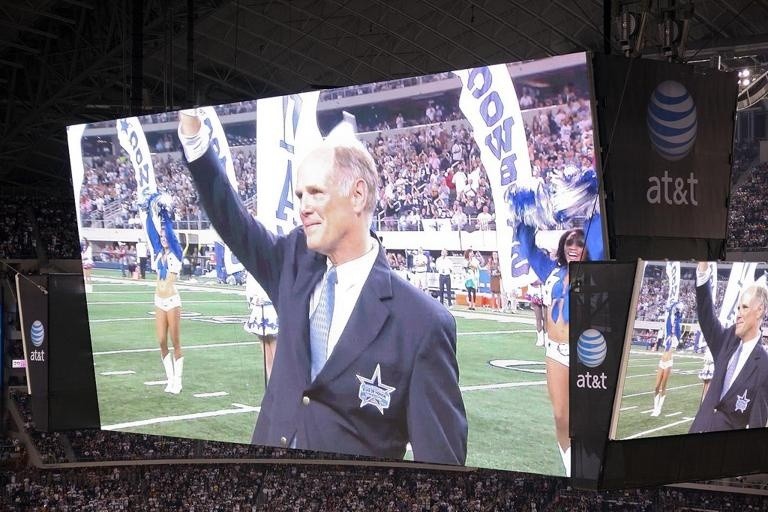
[719,343,743,401]
[308,264,338,384]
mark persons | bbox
[726,135,768,251]
[631,262,768,433]
[2,182,103,511]
[82,58,604,466]
[103,431,602,510]
[602,476,768,511]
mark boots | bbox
[650,392,666,416]
[161,352,184,394]
[536,328,548,348]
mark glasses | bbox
[565,235,585,248]
